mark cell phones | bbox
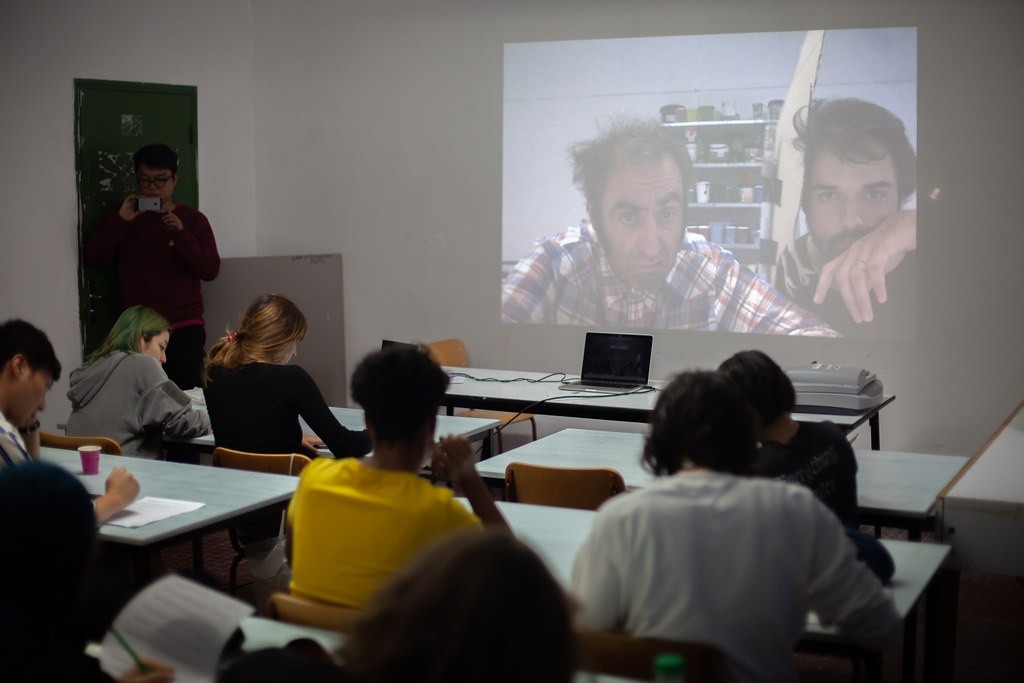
[134,197,162,212]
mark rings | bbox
[853,261,866,271]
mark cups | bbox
[696,181,710,203]
[77,445,102,475]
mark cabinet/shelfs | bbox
[664,118,779,282]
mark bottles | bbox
[768,99,785,120]
[686,189,695,203]
[685,143,697,163]
[653,654,685,683]
[686,222,760,245]
[754,186,764,202]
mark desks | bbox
[240,616,646,683]
[40,446,300,591]
[452,496,953,683]
[474,427,972,683]
[56,388,501,578]
[440,365,897,451]
[935,402,1024,683]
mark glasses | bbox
[139,173,174,190]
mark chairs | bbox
[572,630,737,683]
[422,339,537,454]
[39,431,124,455]
[504,464,625,512]
[269,590,367,633]
[212,446,313,591]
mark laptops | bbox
[559,332,653,394]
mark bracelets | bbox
[19,420,40,435]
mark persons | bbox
[774,96,917,342]
[566,373,897,683]
[202,294,373,551]
[503,117,840,339]
[83,143,221,391]
[64,304,211,460]
[0,319,139,528]
[718,350,858,526]
[284,343,515,619]
[331,531,575,683]
[0,458,178,683]
